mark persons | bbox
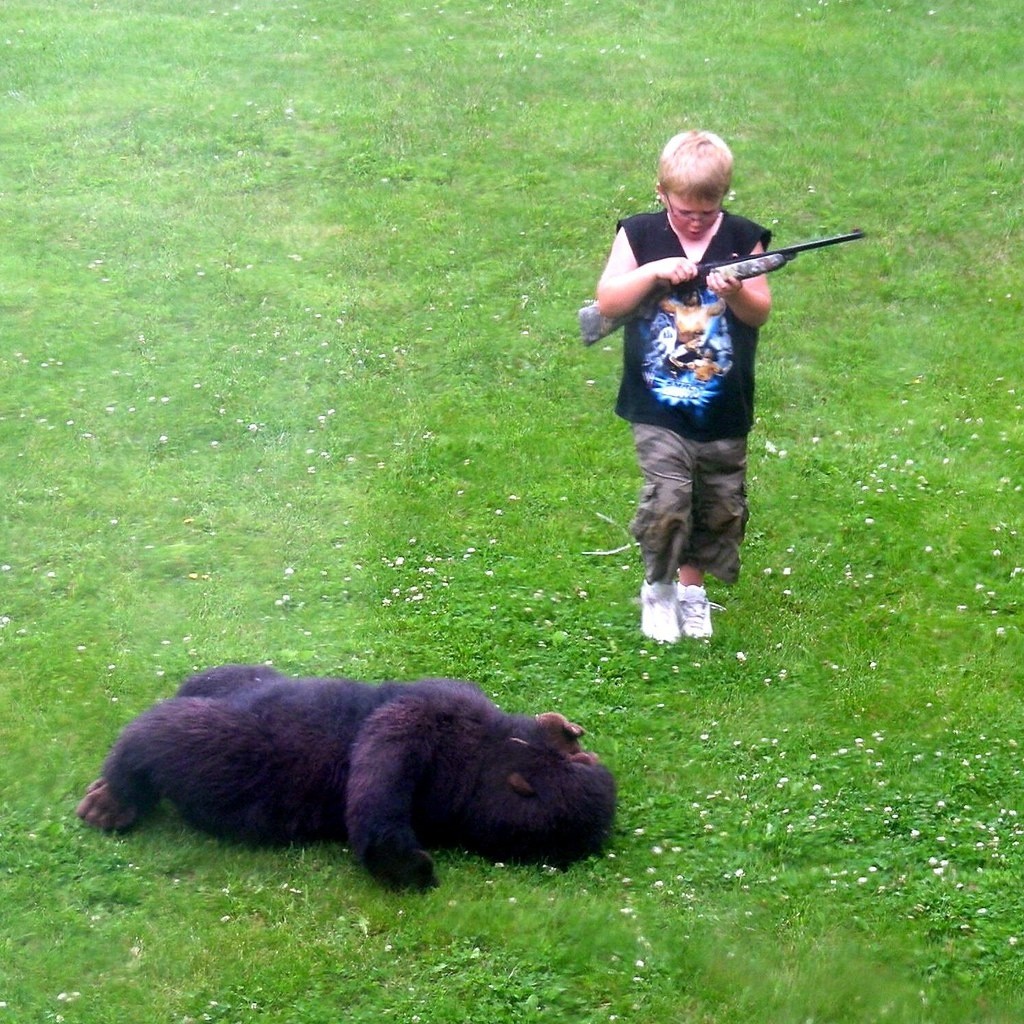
[596,126,774,644]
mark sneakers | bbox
[640,580,685,647]
[677,587,714,639]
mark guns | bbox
[575,226,867,350]
